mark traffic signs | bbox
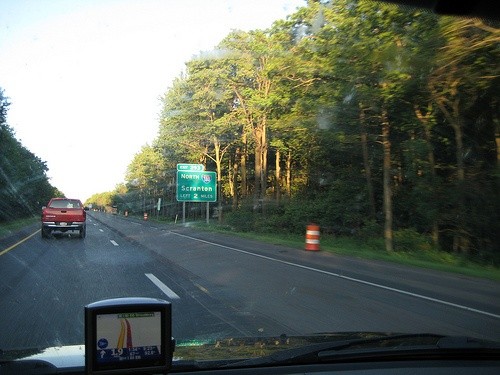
[177,163,205,172]
[176,171,218,203]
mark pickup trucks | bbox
[40,198,89,239]
